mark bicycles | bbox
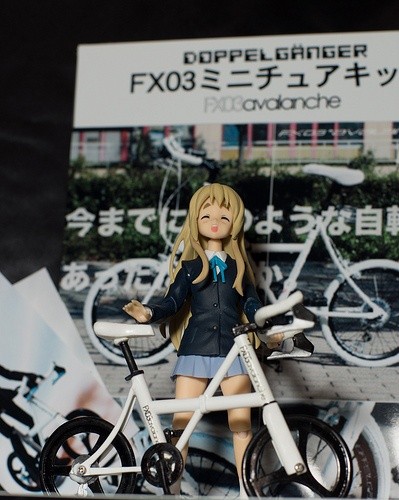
[138,394,392,500]
[82,134,399,367]
[3,362,101,493]
[37,292,355,500]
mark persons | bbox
[121,184,284,497]
[0,365,50,494]
